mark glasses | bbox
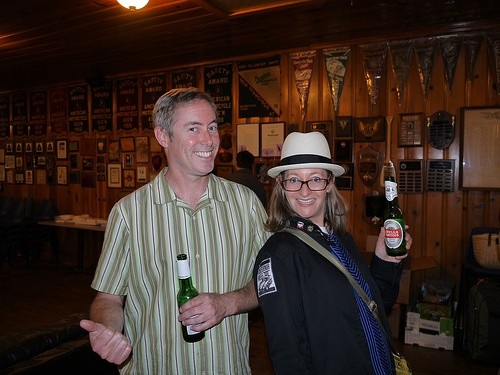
[280,172,332,191]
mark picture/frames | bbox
[397,112,424,147]
[236,123,260,158]
[457,105,500,190]
[259,121,287,159]
[0,137,158,188]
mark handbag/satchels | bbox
[392,351,412,375]
[472,233,500,269]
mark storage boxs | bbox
[405,312,454,350]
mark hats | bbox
[267,132,345,178]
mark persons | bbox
[81,87,275,375]
[254,131,412,375]
[227,150,266,208]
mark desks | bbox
[40,222,106,275]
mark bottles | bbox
[382,175,407,256]
[177,254,204,343]
